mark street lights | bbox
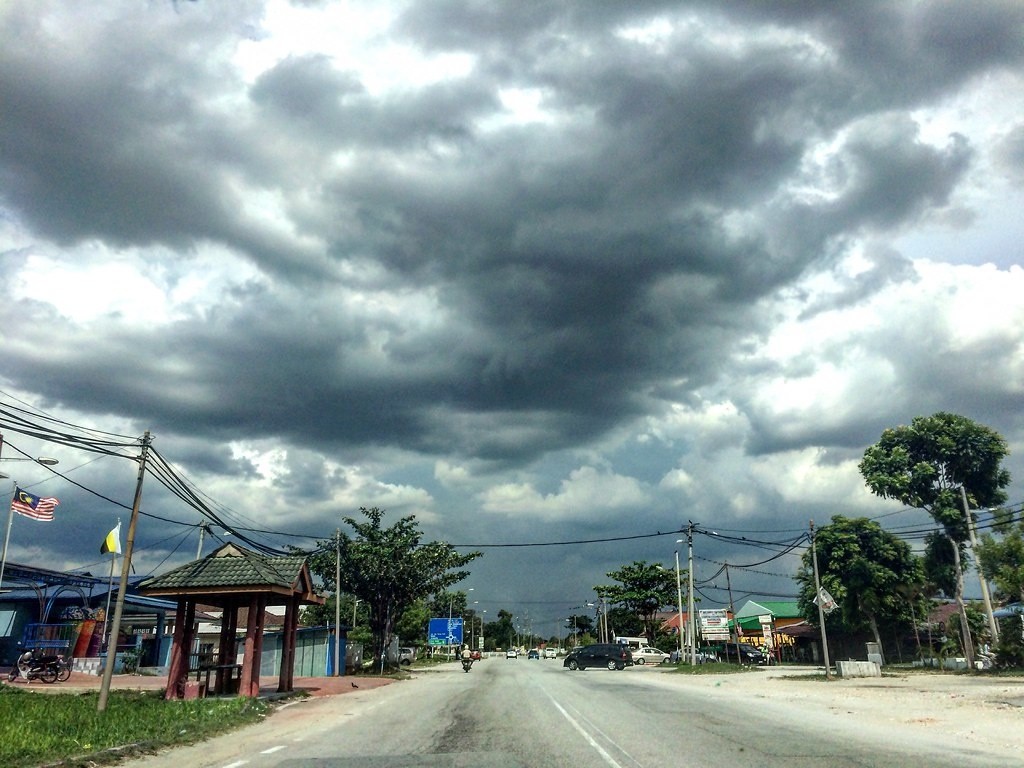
[676,540,696,666]
[587,601,608,644]
[447,589,474,661]
[566,616,576,646]
[966,507,999,644]
[472,611,487,650]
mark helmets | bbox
[464,645,469,650]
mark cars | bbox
[670,647,717,664]
[528,650,539,660]
[632,647,671,666]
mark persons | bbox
[460,645,474,669]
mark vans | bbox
[722,643,766,666]
[563,643,634,672]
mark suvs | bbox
[382,647,417,666]
[543,649,556,659]
[506,649,517,659]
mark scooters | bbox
[461,653,474,673]
[27,647,73,682]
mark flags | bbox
[99,523,122,555]
[11,485,60,522]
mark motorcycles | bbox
[8,641,64,685]
